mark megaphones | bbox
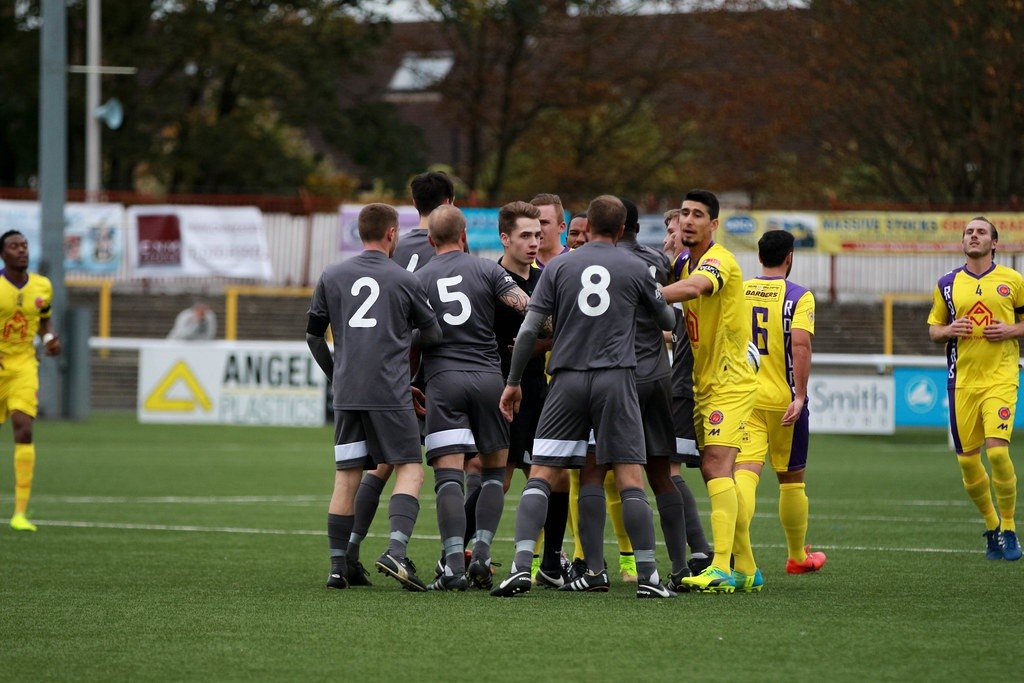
[96,98,123,130]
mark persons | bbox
[742,230,827,574]
[163,294,218,340]
[928,216,1024,561]
[305,172,764,599]
[0,230,62,532]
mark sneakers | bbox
[426,572,468,591]
[346,560,372,586]
[536,567,572,590]
[558,549,572,571]
[637,570,677,598]
[557,572,609,592]
[983,524,1002,558]
[665,566,693,592]
[465,549,502,575]
[531,557,545,586]
[730,567,763,593]
[466,558,493,592]
[682,566,736,594]
[10,513,37,532]
[998,530,1022,561]
[618,554,638,583]
[327,571,351,590]
[688,551,734,576]
[435,557,448,574]
[374,550,427,592]
[568,557,607,582]
[787,543,826,574]
[489,567,531,597]
